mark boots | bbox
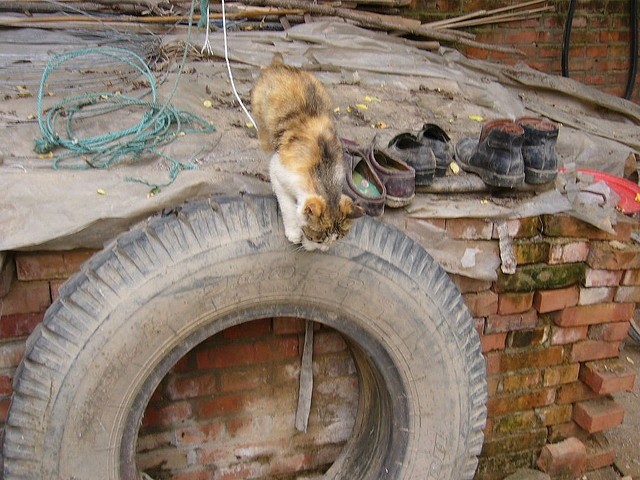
[516,117,559,184]
[454,120,525,188]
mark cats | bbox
[251,53,367,252]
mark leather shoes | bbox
[342,145,386,218]
[418,124,454,176]
[340,137,415,209]
[386,133,436,186]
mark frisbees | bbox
[559,168,640,214]
